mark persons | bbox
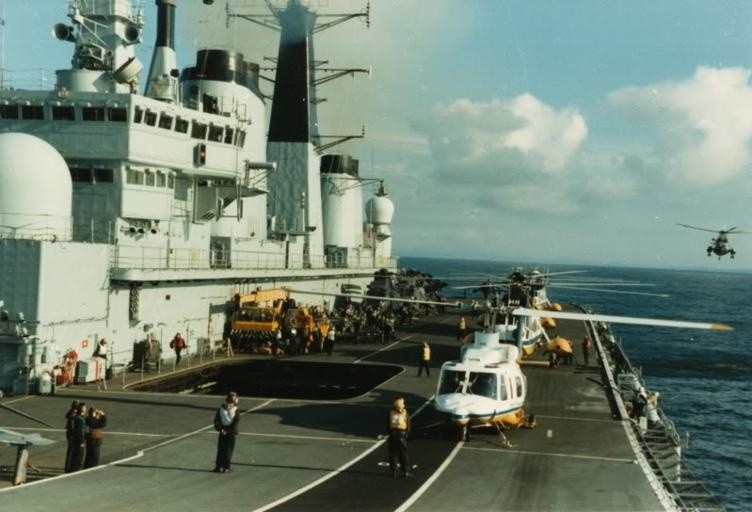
[416,338,432,378]
[213,389,240,475]
[63,397,107,473]
[93,338,111,391]
[629,385,661,427]
[311,302,415,357]
[580,335,591,365]
[169,332,188,368]
[382,397,414,479]
[456,315,467,341]
[547,338,574,369]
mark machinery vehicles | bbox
[231,286,333,357]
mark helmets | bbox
[226,392,238,404]
[393,398,405,409]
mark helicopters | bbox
[676,223,751,259]
[282,266,734,442]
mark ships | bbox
[1,0,727,512]
